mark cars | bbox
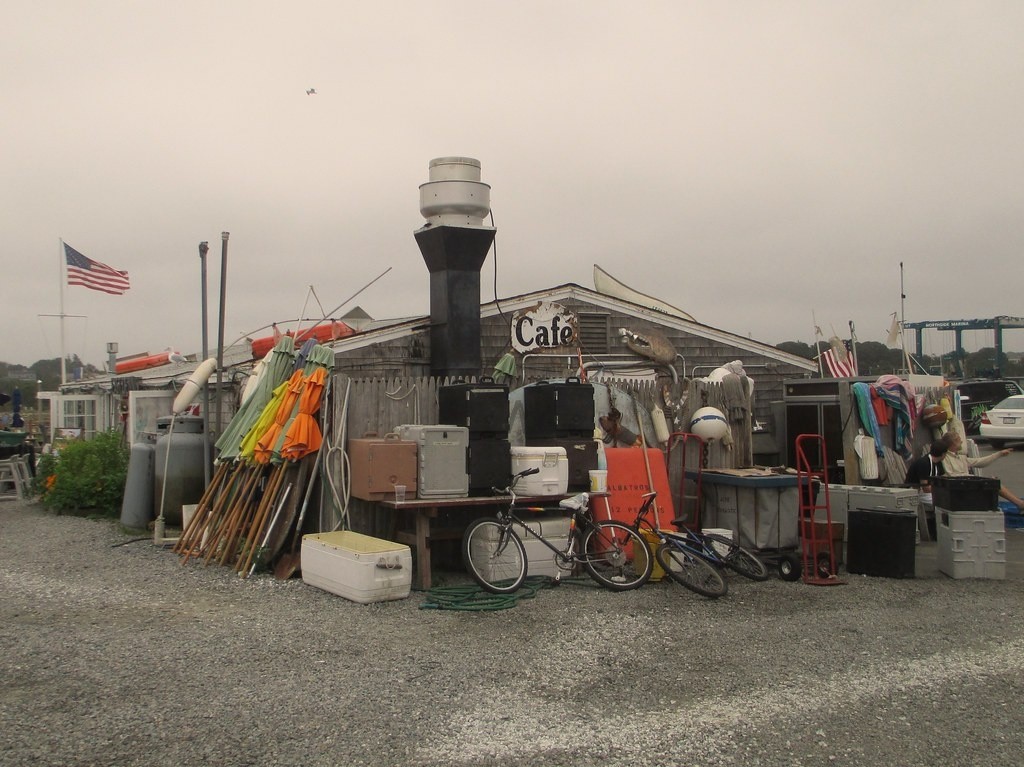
[978,394,1024,449]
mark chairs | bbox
[880,445,935,543]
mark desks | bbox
[378,492,612,591]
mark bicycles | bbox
[617,490,769,598]
[460,467,654,595]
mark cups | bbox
[588,470,608,492]
[394,485,406,504]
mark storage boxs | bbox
[930,475,1002,512]
[510,445,569,496]
[999,501,1024,528]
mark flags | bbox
[823,341,856,378]
[63,241,130,295]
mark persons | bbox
[904,440,949,505]
[942,431,1024,514]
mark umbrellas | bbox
[173,327,336,578]
[13,387,23,432]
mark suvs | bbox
[945,375,1024,436]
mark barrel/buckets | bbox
[919,491,932,505]
[670,532,688,572]
[701,528,734,563]
